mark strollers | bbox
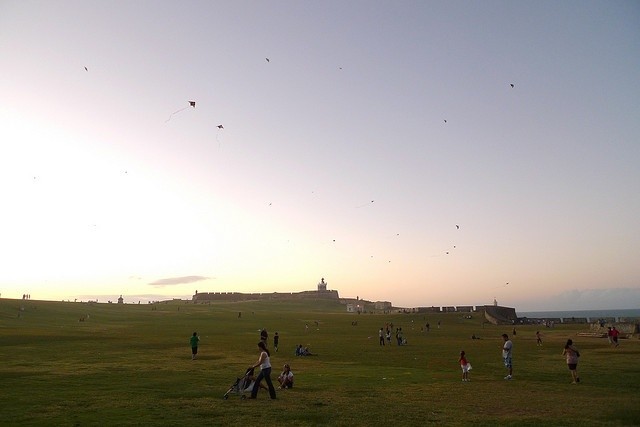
[223,365,254,399]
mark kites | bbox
[188,101,196,108]
[217,125,225,129]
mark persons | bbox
[189,332,200,360]
[512,327,517,337]
[242,367,268,392]
[260,327,269,348]
[456,350,471,381]
[420,325,424,333]
[388,322,394,329]
[411,318,414,323]
[562,338,580,385]
[273,332,279,351]
[296,344,306,354]
[385,325,390,332]
[511,318,555,328]
[277,364,294,389]
[295,344,306,356]
[385,330,392,346]
[395,326,408,345]
[536,331,544,347]
[247,342,276,399]
[612,326,619,343]
[606,326,615,345]
[378,327,385,345]
[463,315,472,319]
[304,343,318,355]
[437,319,441,328]
[426,321,430,331]
[501,334,513,379]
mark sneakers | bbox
[462,378,470,381]
[503,375,511,379]
